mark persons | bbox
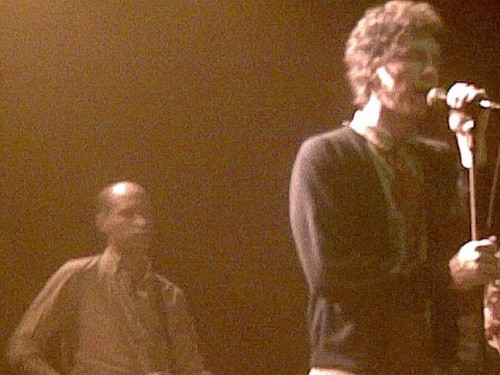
[2,179,209,375]
[288,1,500,375]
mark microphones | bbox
[426,88,499,112]
